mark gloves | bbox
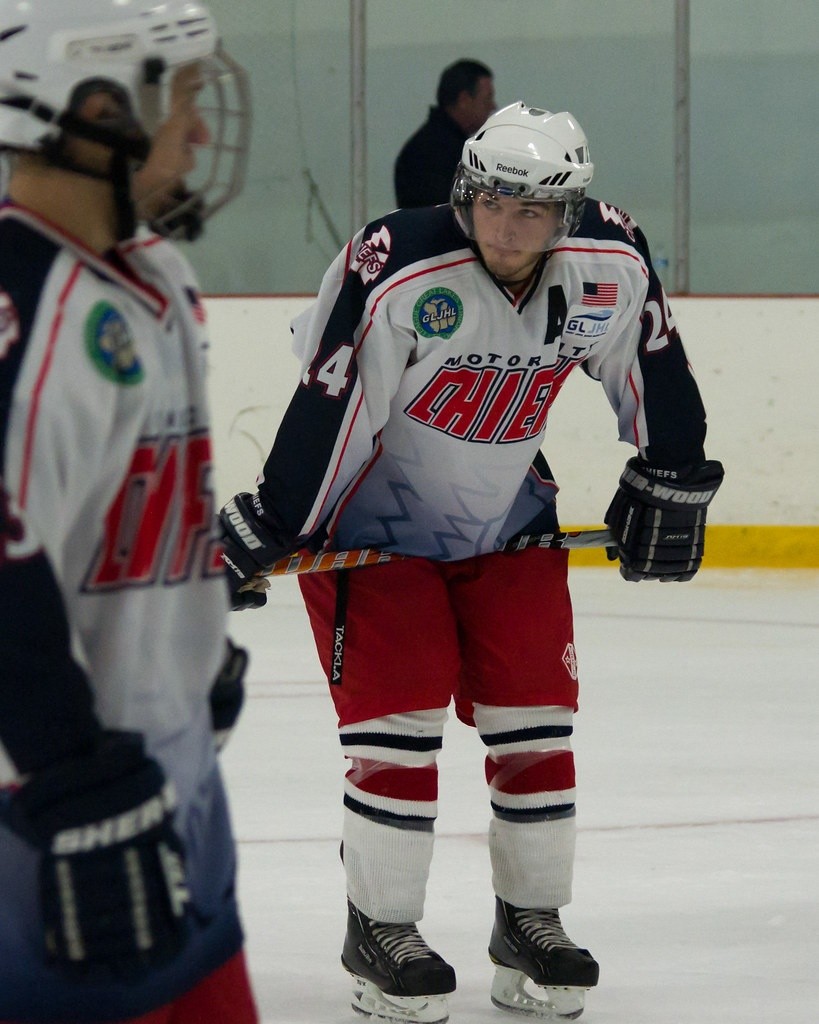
[605,453,726,582]
[30,747,201,973]
[215,487,277,612]
[205,643,247,756]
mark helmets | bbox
[0,0,249,237]
[453,98,594,254]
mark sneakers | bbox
[339,900,458,1023]
[489,894,599,1019]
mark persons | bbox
[217,99,725,997]
[0,0,259,1024]
[389,57,504,213]
